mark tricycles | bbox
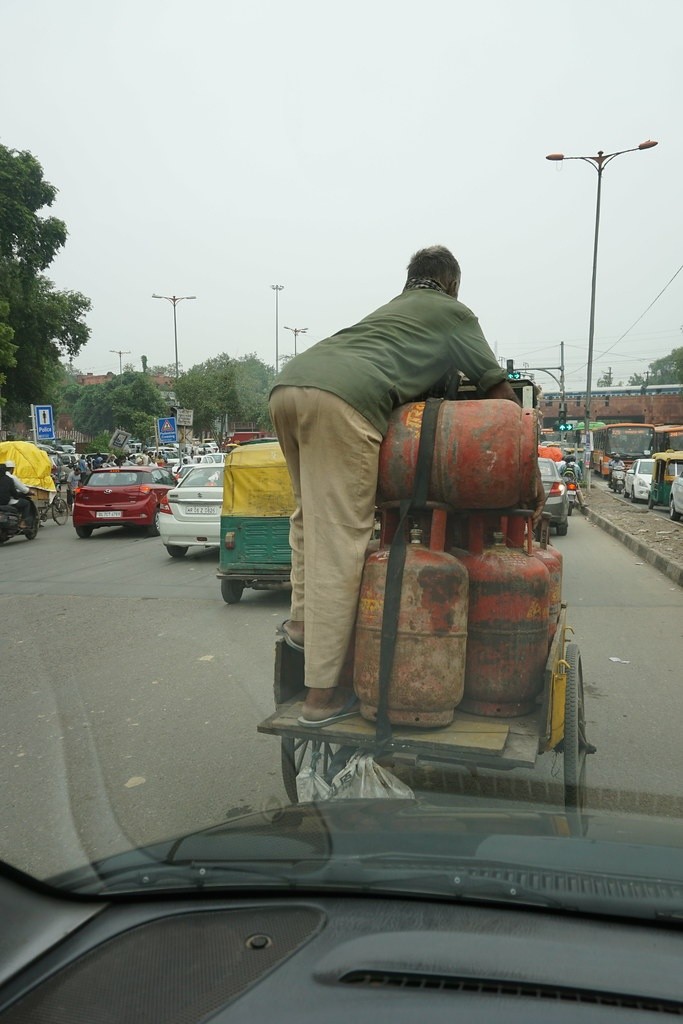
[0,440,69,526]
[257,531,599,836]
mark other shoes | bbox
[581,503,589,508]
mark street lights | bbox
[110,350,132,375]
[272,283,285,373]
[151,294,196,380]
[545,140,658,419]
[283,326,309,358]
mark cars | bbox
[26,431,266,486]
[537,457,571,535]
[669,471,683,521]
[71,466,180,538]
[158,463,225,559]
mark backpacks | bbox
[562,463,576,481]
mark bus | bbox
[540,422,683,515]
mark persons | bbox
[607,452,626,487]
[72,441,76,448]
[78,447,168,483]
[558,455,589,508]
[7,431,14,441]
[665,442,677,451]
[50,464,82,516]
[182,450,201,466]
[268,244,546,728]
[0,460,36,529]
[25,434,30,440]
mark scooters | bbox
[0,490,40,545]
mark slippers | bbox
[298,694,360,728]
[276,619,304,653]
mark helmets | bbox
[611,452,615,455]
[565,456,576,463]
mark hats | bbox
[6,462,15,467]
[615,454,620,457]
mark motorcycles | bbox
[647,449,683,509]
[216,440,295,605]
